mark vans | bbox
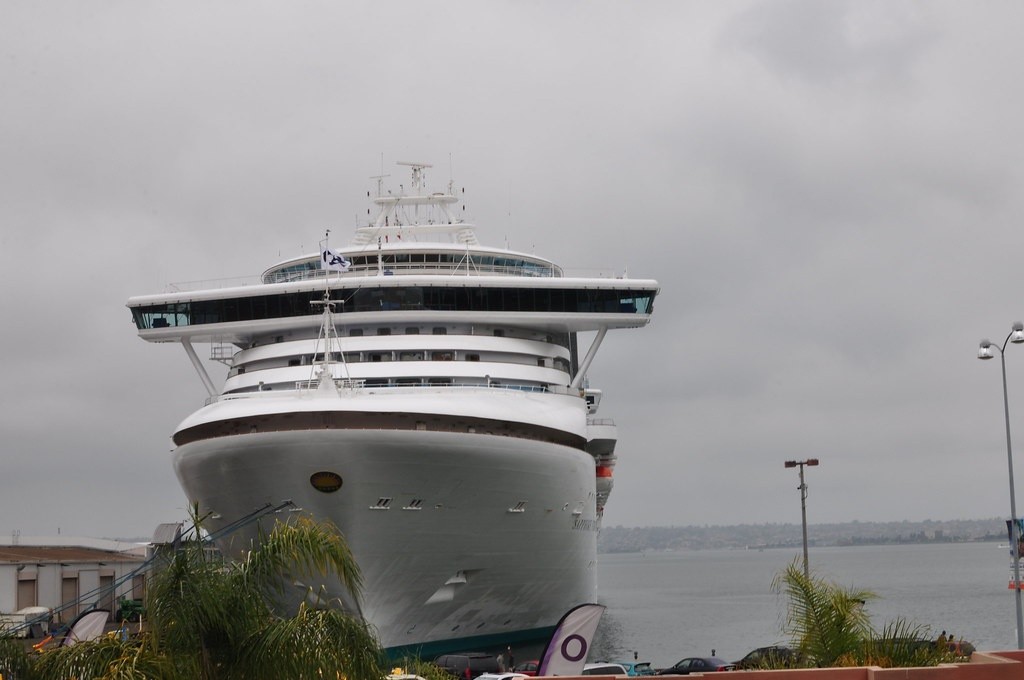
[432,653,501,680]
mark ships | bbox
[124,155,662,667]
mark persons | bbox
[934,630,955,662]
[495,646,516,673]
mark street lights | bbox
[784,459,819,621]
[977,321,1023,650]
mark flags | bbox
[319,243,351,271]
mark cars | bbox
[582,663,629,675]
[472,672,530,680]
[730,645,794,670]
[512,661,541,677]
[657,657,737,675]
[615,662,657,676]
